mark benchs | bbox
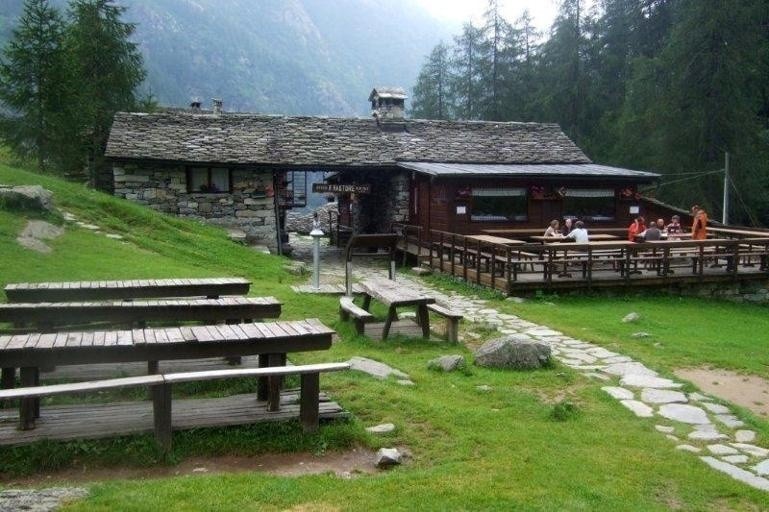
[339,295,372,335]
[429,303,462,345]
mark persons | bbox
[633,221,661,253]
[312,212,322,230]
[690,205,709,264]
[655,217,667,233]
[558,219,575,235]
[560,221,590,252]
[627,215,647,242]
[667,215,688,261]
[542,219,563,244]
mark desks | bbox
[358,277,435,343]
